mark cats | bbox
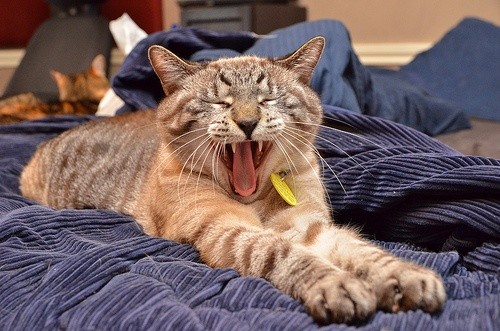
[0,54,111,125]
[19,35,446,320]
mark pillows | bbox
[0,15,113,104]
